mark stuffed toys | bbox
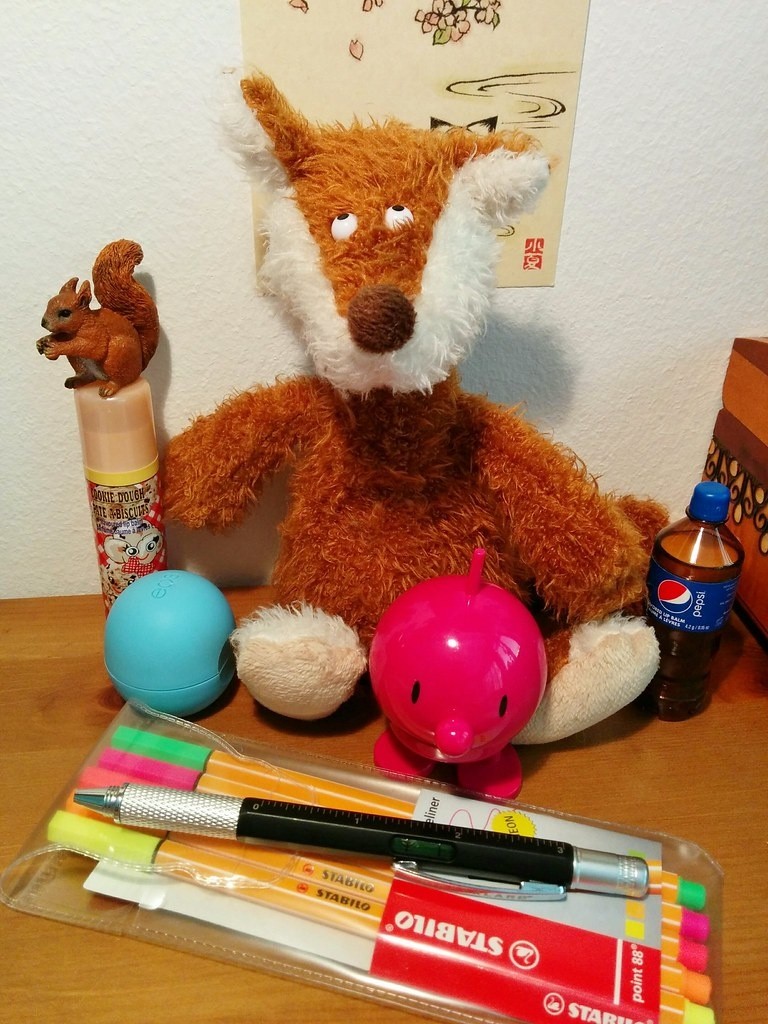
[156,69,672,748]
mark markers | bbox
[45,724,719,1024]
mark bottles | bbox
[642,482,744,721]
[79,381,167,614]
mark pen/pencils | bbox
[72,782,649,904]
